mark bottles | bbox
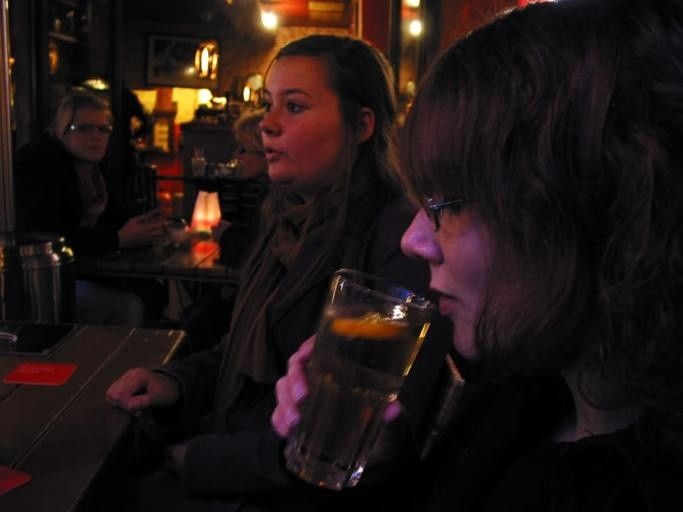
[166,218,191,246]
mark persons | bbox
[106,30,415,502]
[15,86,177,332]
[272,2,683,511]
[181,106,270,264]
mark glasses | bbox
[68,122,113,135]
[412,189,465,233]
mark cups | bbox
[284,268,439,492]
[206,163,224,177]
[190,156,205,177]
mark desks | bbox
[0,321,188,512]
[86,222,262,346]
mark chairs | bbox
[415,353,466,463]
[185,177,261,240]
